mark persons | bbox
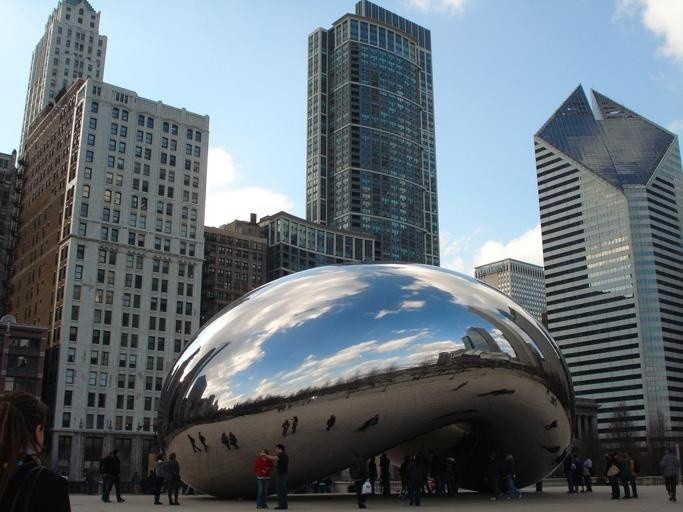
[295,442,522,508]
[85,448,181,506]
[252,449,273,509]
[229,432,239,450]
[197,431,207,453]
[259,445,289,510]
[281,420,289,437]
[564,450,639,500]
[358,415,381,432]
[0,389,70,512]
[325,415,336,433]
[658,447,681,502]
[291,415,298,433]
[187,434,201,456]
[535,480,543,492]
[219,433,229,450]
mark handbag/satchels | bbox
[606,465,619,477]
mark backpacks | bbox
[629,458,639,472]
